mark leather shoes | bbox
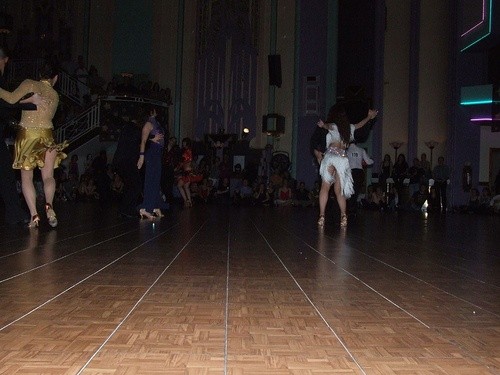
[117,210,134,218]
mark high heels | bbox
[183,199,187,209]
[340,212,347,231]
[317,216,325,228]
[140,208,156,221]
[45,203,58,228]
[189,200,192,208]
[24,215,40,228]
[153,208,164,217]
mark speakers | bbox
[268,55,282,88]
